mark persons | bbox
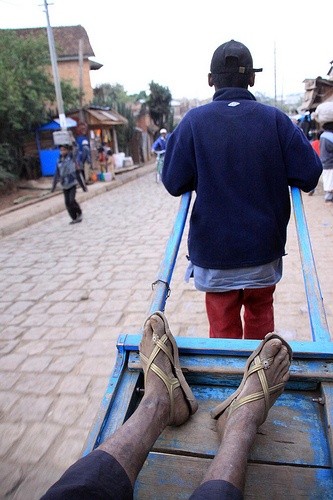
[81,139,117,184]
[51,144,88,225]
[158,39,324,339]
[38,311,293,500]
[151,129,169,159]
[293,107,333,201]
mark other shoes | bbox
[325,198,333,202]
[76,216,82,223]
[69,220,77,224]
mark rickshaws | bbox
[74,177,333,500]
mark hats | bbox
[59,144,72,150]
[160,129,167,134]
[210,39,263,75]
[82,140,88,145]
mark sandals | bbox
[211,333,293,442]
[139,311,198,426]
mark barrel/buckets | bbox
[90,172,112,181]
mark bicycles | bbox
[153,149,166,184]
